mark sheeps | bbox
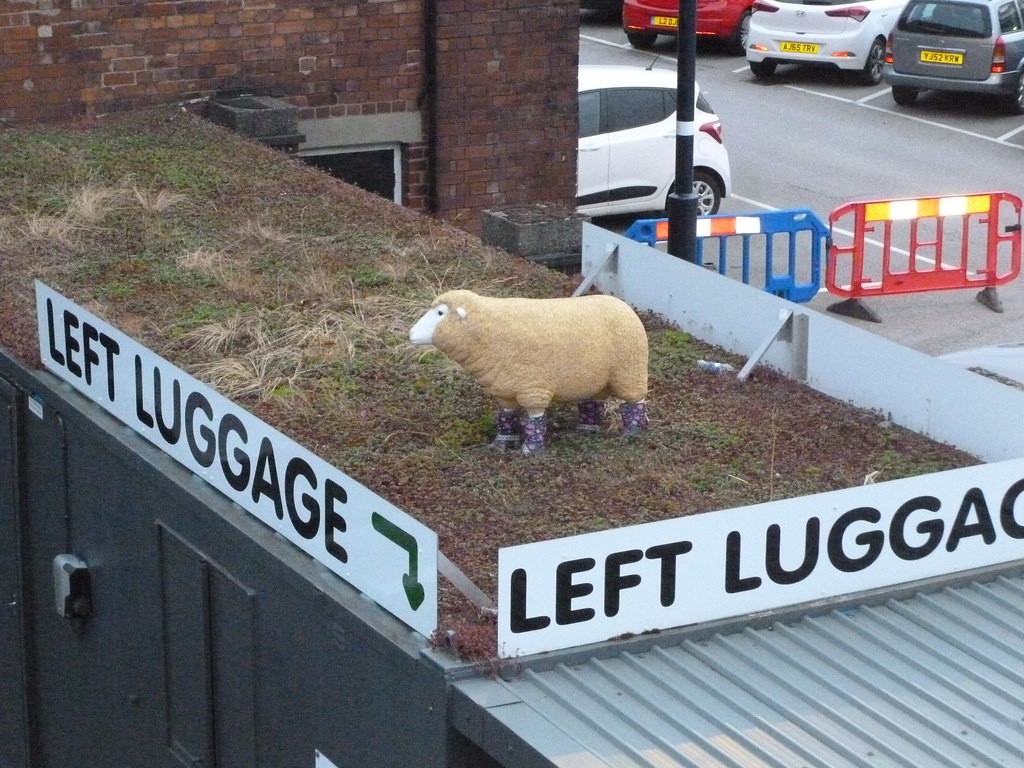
[407,288,649,456]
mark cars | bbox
[623,0,752,56]
[745,0,937,85]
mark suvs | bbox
[883,0,1024,116]
[574,65,731,222]
